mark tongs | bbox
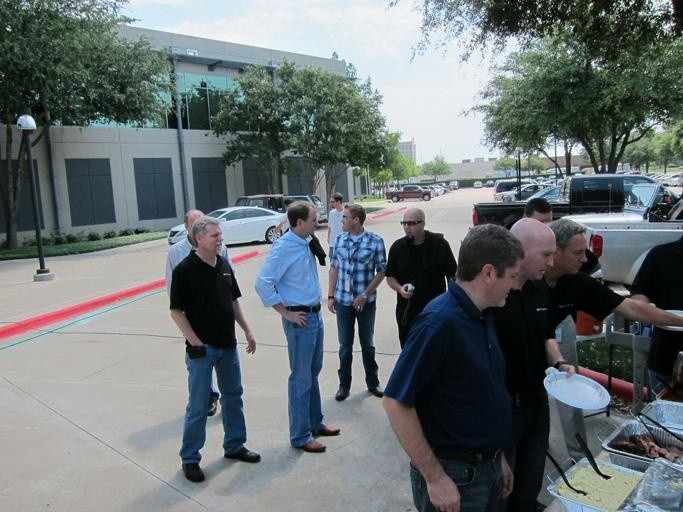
[637,411,683,447]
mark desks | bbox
[540,440,614,512]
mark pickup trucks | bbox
[383,185,432,201]
[468,174,672,236]
[558,197,682,294]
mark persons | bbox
[276,198,297,235]
[169,215,261,482]
[327,192,348,263]
[165,209,236,416]
[328,204,387,401]
[255,200,340,451]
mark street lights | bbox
[14,113,55,281]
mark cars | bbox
[162,206,292,247]
[419,183,453,197]
[473,181,483,188]
[493,172,682,206]
[485,180,494,188]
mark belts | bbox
[434,447,503,463]
[286,303,321,312]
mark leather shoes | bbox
[300,440,326,452]
[182,463,204,482]
[225,447,260,462]
[368,385,384,396]
[318,425,340,435]
[336,388,349,400]
[208,400,216,416]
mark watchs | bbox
[361,292,369,299]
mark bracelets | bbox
[328,296,334,301]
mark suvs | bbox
[447,181,461,191]
[235,193,286,214]
[283,194,327,226]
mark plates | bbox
[654,309,682,331]
[543,366,611,410]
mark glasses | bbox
[401,221,422,225]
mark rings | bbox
[358,306,360,309]
